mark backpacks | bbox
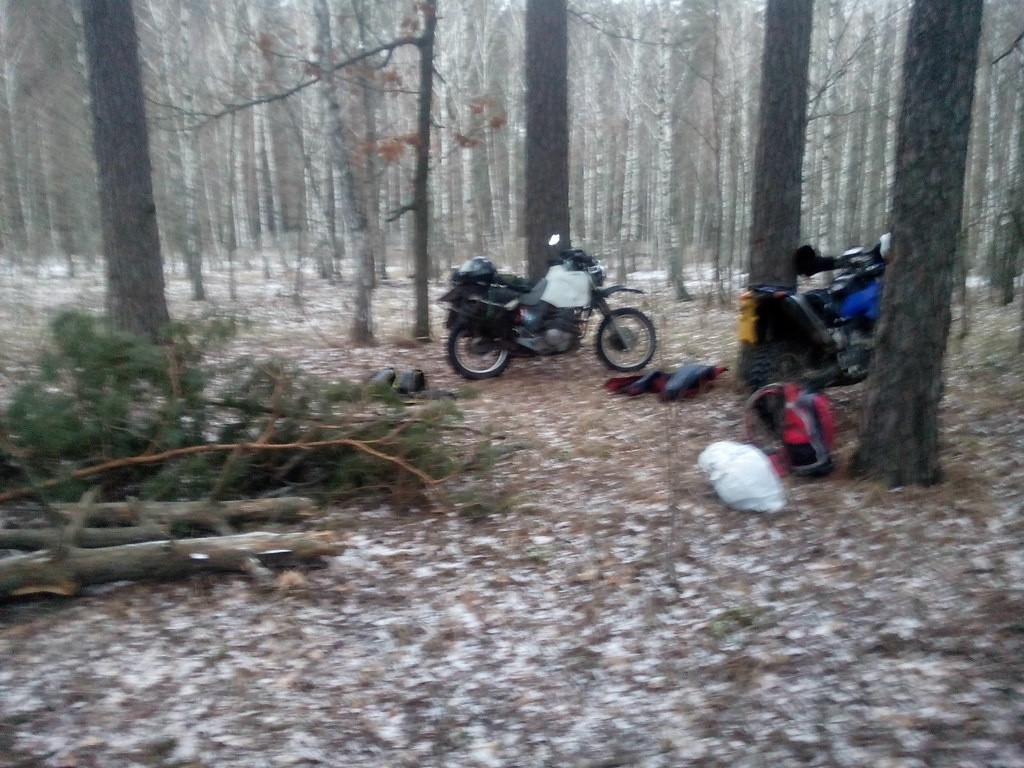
[743,380,837,479]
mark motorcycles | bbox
[741,244,879,398]
[438,235,656,380]
